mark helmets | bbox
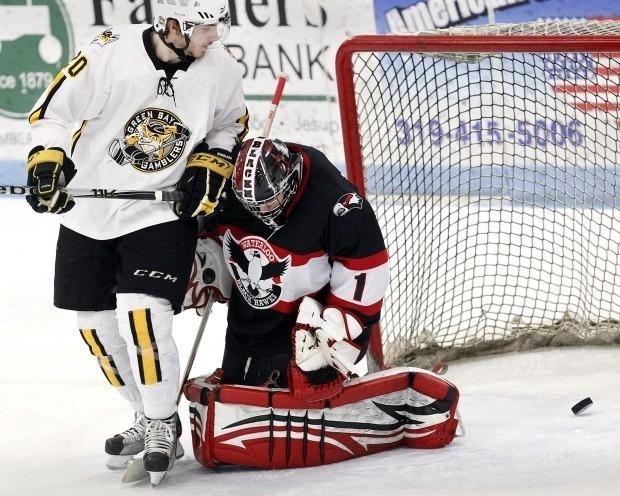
[149,0,231,34]
[232,134,304,207]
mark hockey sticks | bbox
[121,72,289,485]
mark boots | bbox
[103,413,183,457]
[144,414,177,473]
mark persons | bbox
[24,0,252,486]
[180,136,464,471]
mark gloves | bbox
[25,142,77,216]
[178,145,233,220]
